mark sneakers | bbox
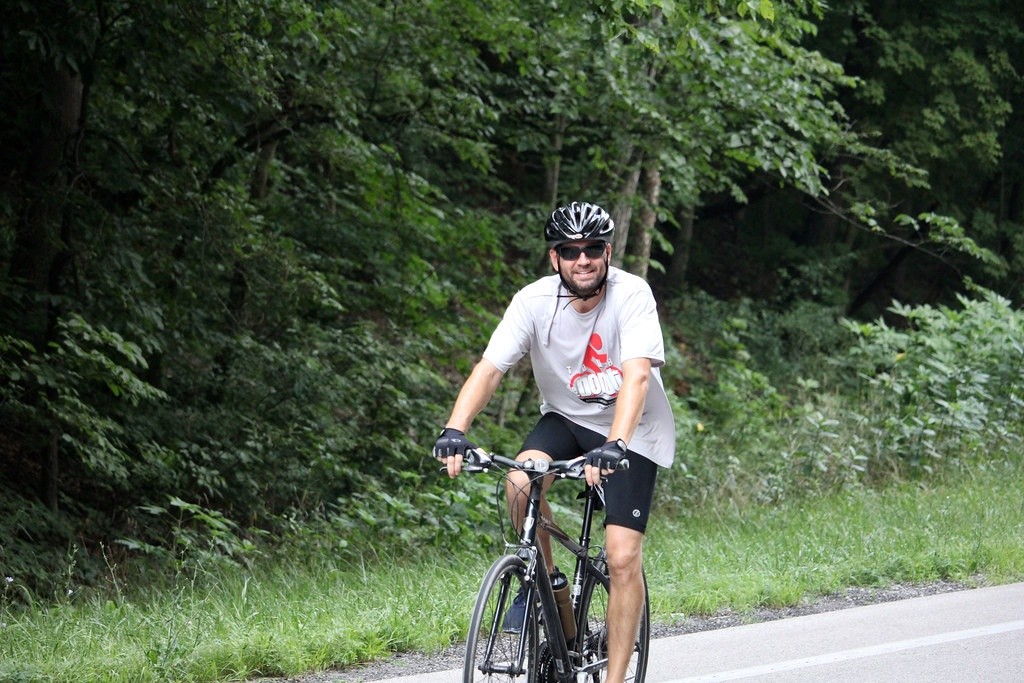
[501,588,526,634]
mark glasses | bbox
[554,242,605,260]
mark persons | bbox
[432,202,676,683]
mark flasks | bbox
[549,565,577,641]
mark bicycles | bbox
[439,447,652,683]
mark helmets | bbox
[544,201,615,249]
